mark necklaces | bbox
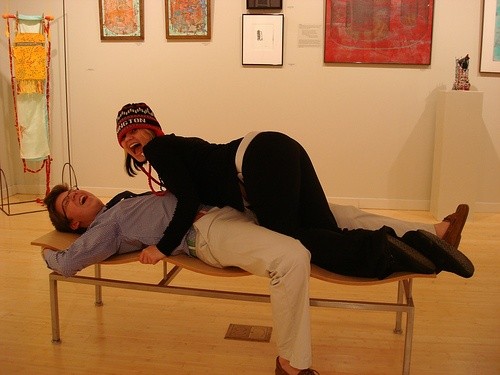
[159,162,167,194]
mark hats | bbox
[116,102,169,196]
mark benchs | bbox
[31,229,436,375]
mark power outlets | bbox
[225,324,273,342]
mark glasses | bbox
[62,185,79,220]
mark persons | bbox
[116,103,474,279]
[41,184,469,375]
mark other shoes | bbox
[442,204,470,249]
[417,229,475,278]
[275,356,320,375]
[387,234,436,274]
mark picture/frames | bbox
[165,0,212,39]
[99,0,144,40]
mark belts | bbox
[186,227,197,257]
[235,131,262,183]
